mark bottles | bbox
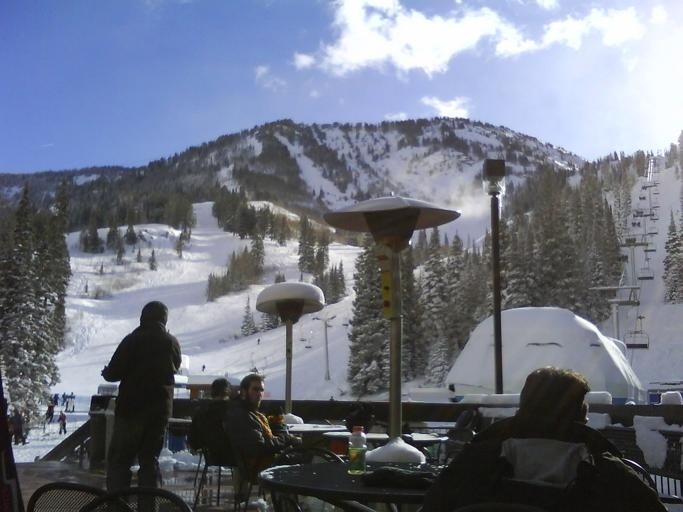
[347,425,369,474]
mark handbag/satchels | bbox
[186,400,208,452]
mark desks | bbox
[324,432,450,449]
[258,462,427,512]
[284,421,347,435]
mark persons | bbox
[100,300,183,511]
[418,364,671,512]
[7,408,30,446]
[57,411,67,436]
[41,402,55,425]
[38,391,76,413]
[224,373,313,487]
[185,377,249,504]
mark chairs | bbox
[26,482,191,511]
[621,457,656,489]
[190,416,304,512]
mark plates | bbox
[372,462,443,480]
